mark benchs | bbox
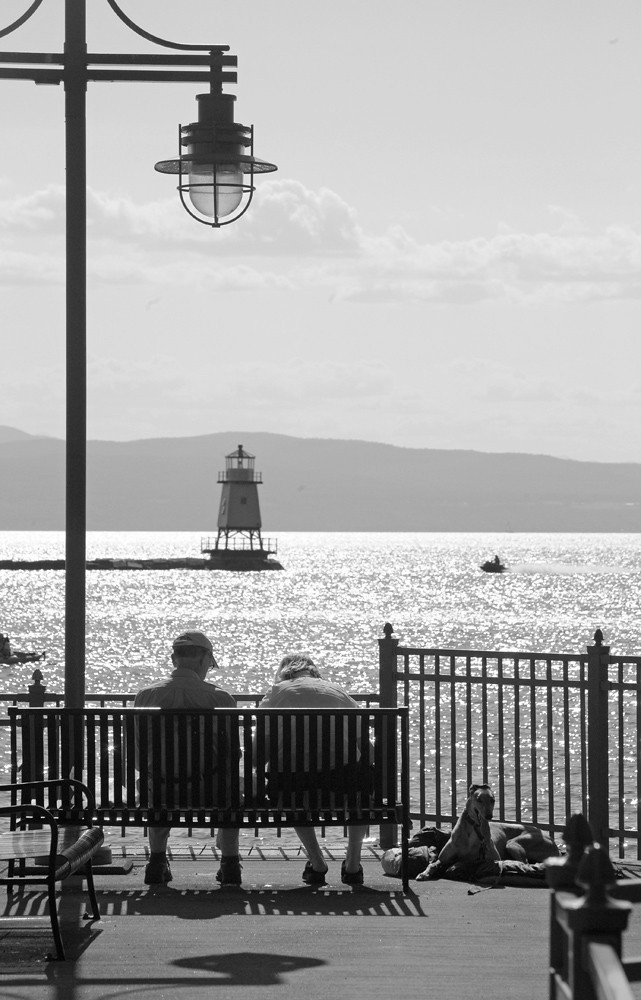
[0,780,105,964]
[8,704,417,894]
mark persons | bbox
[251,654,375,884]
[125,632,245,885]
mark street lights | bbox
[0,0,278,853]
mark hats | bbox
[173,631,219,669]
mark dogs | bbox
[382,783,559,888]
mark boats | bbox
[480,561,504,573]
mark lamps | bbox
[153,76,276,224]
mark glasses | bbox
[206,651,214,672]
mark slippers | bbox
[302,861,326,884]
[341,859,363,884]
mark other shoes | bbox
[216,858,242,885]
[145,860,172,884]
